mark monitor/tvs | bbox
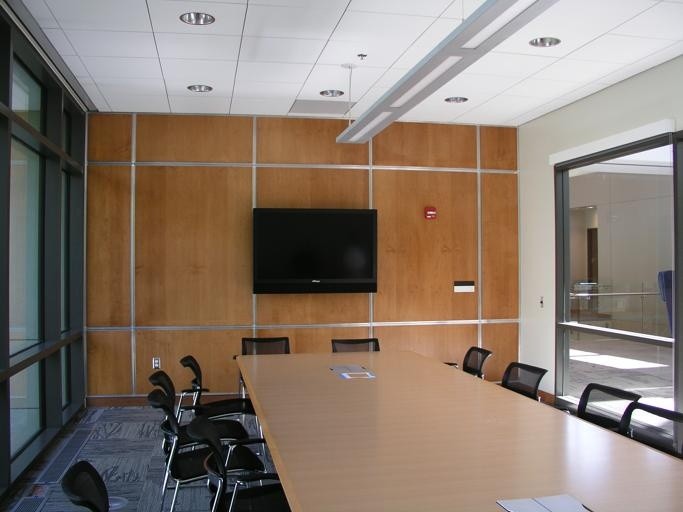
[252,207,378,294]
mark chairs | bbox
[444,346,682,461]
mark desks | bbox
[235,349,683,512]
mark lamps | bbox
[335,0,561,146]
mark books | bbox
[496,494,591,512]
